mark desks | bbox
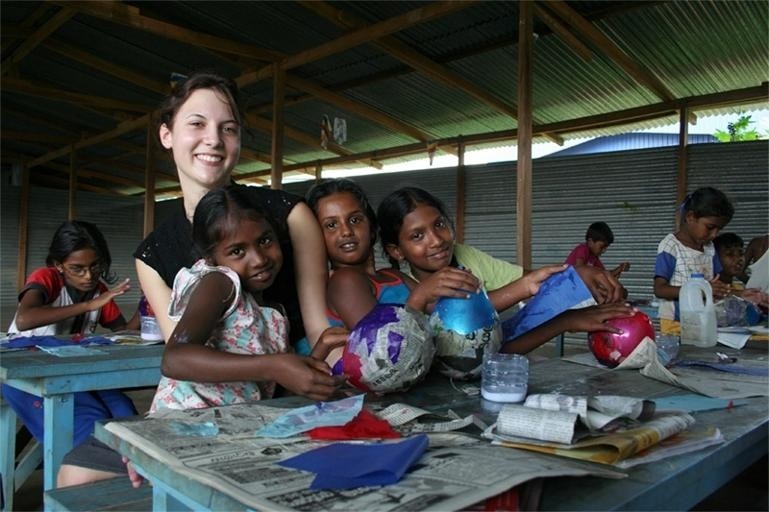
[0,332,163,486]
[92,319,768,510]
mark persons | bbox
[296,178,567,359]
[562,221,629,282]
[379,186,639,355]
[0,219,140,451]
[653,187,766,337]
[150,186,346,415]
[713,233,769,310]
[744,235,769,277]
[56,73,345,494]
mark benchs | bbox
[46,476,153,512]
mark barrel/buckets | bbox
[679,273,717,348]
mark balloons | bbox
[726,300,763,325]
[341,303,435,393]
[429,264,502,380]
[590,309,655,369]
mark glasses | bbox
[55,263,105,277]
